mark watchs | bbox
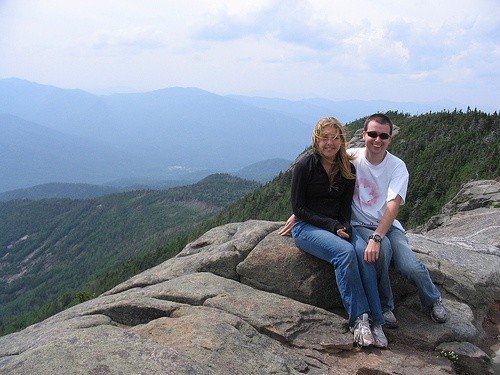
[369,235,383,243]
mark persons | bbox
[291,117,388,348]
[278,114,448,328]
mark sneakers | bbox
[383,311,398,328]
[430,301,447,323]
[349,313,374,347]
[370,325,388,349]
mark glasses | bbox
[366,131,391,139]
